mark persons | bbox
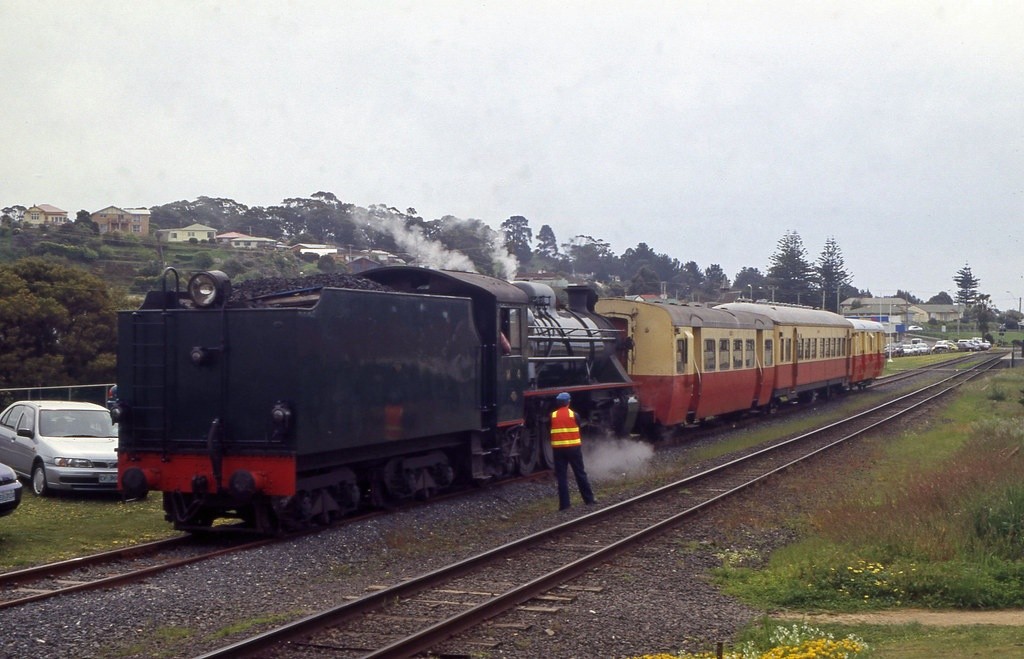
[546,392,600,511]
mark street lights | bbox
[1005,290,1021,331]
[748,284,752,303]
[887,302,897,362]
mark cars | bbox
[0,399,119,498]
[908,325,923,330]
[0,462,21,517]
[885,337,992,358]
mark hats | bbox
[556,392,571,406]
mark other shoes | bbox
[589,498,598,505]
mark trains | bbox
[106,261,887,538]
